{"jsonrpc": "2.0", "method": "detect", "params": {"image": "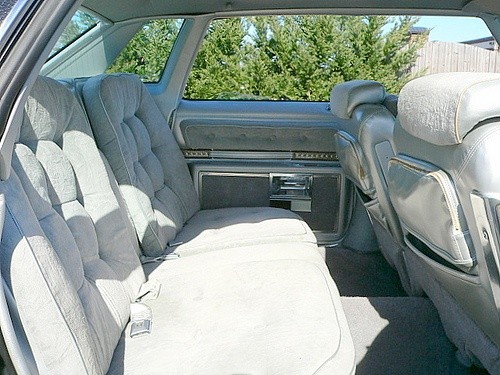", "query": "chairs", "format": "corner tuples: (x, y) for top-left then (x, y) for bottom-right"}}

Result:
(0, 74), (500, 375)
